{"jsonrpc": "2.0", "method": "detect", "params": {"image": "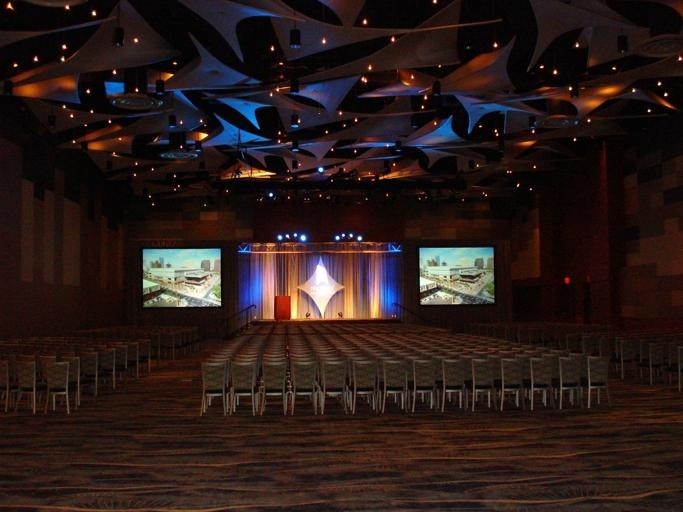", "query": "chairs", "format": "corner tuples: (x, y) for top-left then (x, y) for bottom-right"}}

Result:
(198, 319), (615, 417)
(2, 319), (201, 418)
(463, 319), (682, 393)
